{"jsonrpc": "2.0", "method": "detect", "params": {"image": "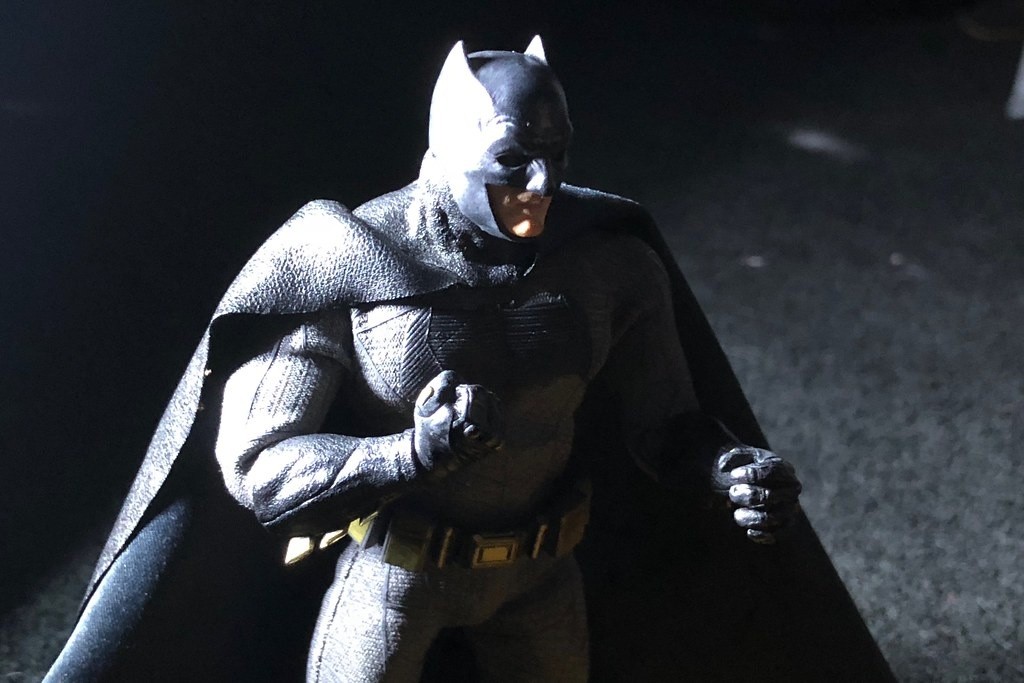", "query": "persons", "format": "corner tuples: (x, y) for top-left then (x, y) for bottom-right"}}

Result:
(41, 33), (899, 683)
